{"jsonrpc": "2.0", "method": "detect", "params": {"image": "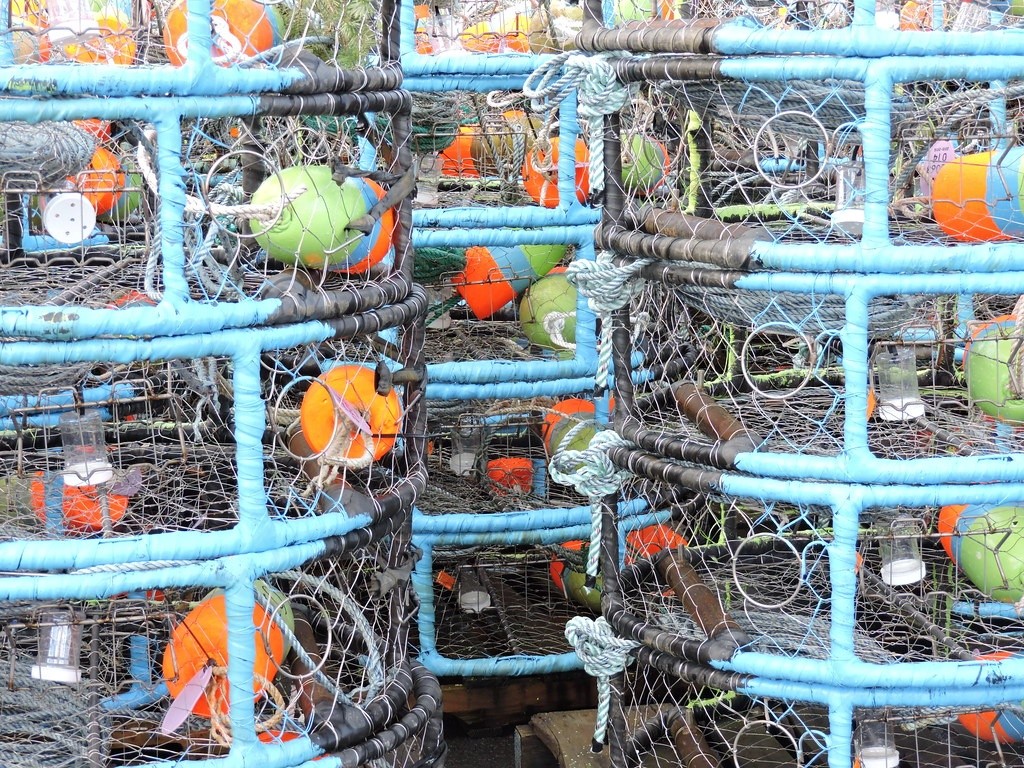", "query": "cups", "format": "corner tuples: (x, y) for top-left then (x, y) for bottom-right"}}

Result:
(425, 282), (452, 329)
(32, 609), (87, 683)
(61, 411), (112, 486)
(40, 179), (97, 243)
(875, 347), (924, 420)
(458, 565), (491, 616)
(427, 15), (464, 55)
(831, 161), (865, 233)
(45, 0), (100, 45)
(416, 153), (444, 204)
(447, 419), (483, 476)
(855, 707), (902, 768)
(874, 514), (926, 586)
